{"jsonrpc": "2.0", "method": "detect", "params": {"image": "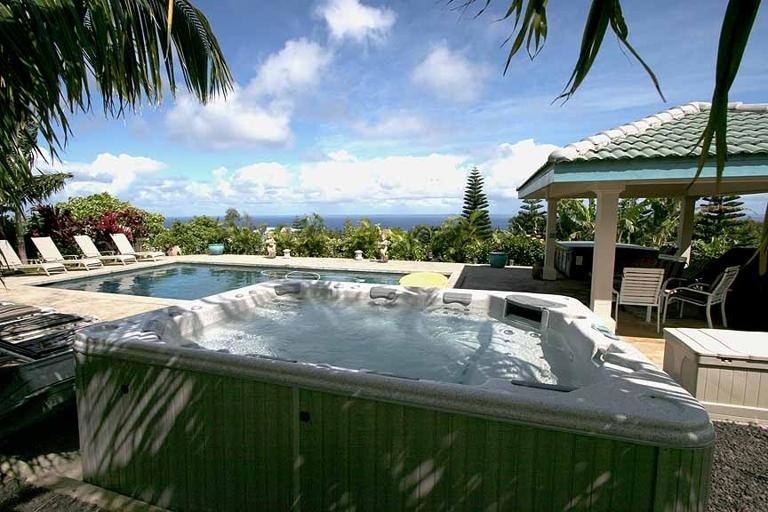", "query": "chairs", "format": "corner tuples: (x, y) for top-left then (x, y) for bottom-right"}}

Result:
(661, 266), (739, 329)
(31, 236), (105, 270)
(0, 240), (69, 276)
(73, 235), (138, 265)
(0, 300), (102, 370)
(662, 248), (761, 312)
(589, 267), (665, 333)
(110, 233), (166, 262)
